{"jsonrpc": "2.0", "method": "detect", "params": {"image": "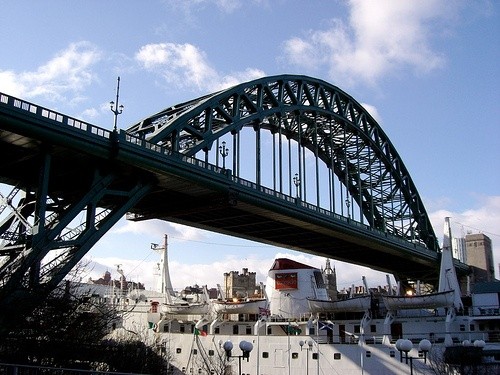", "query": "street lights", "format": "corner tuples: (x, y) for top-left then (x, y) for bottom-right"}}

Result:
(461, 338), (486, 375)
(110, 76), (124, 136)
(224, 339), (253, 375)
(219, 141), (229, 170)
(396, 338), (431, 375)
(80, 215), (500, 375)
(292, 173), (301, 199)
(300, 340), (313, 375)
